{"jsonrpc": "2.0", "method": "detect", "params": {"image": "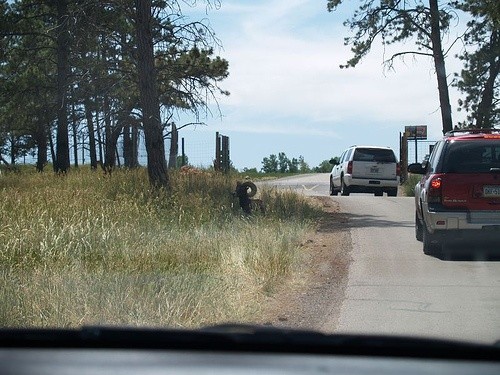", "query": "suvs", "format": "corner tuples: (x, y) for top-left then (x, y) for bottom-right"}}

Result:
(329, 145), (400, 197)
(405, 128), (500, 257)
